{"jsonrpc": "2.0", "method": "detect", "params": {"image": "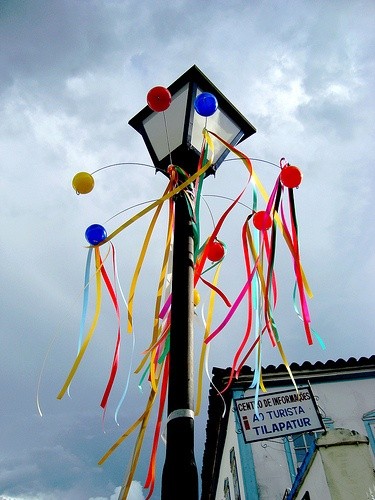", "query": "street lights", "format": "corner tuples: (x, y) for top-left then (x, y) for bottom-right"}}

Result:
(126, 65), (255, 500)
